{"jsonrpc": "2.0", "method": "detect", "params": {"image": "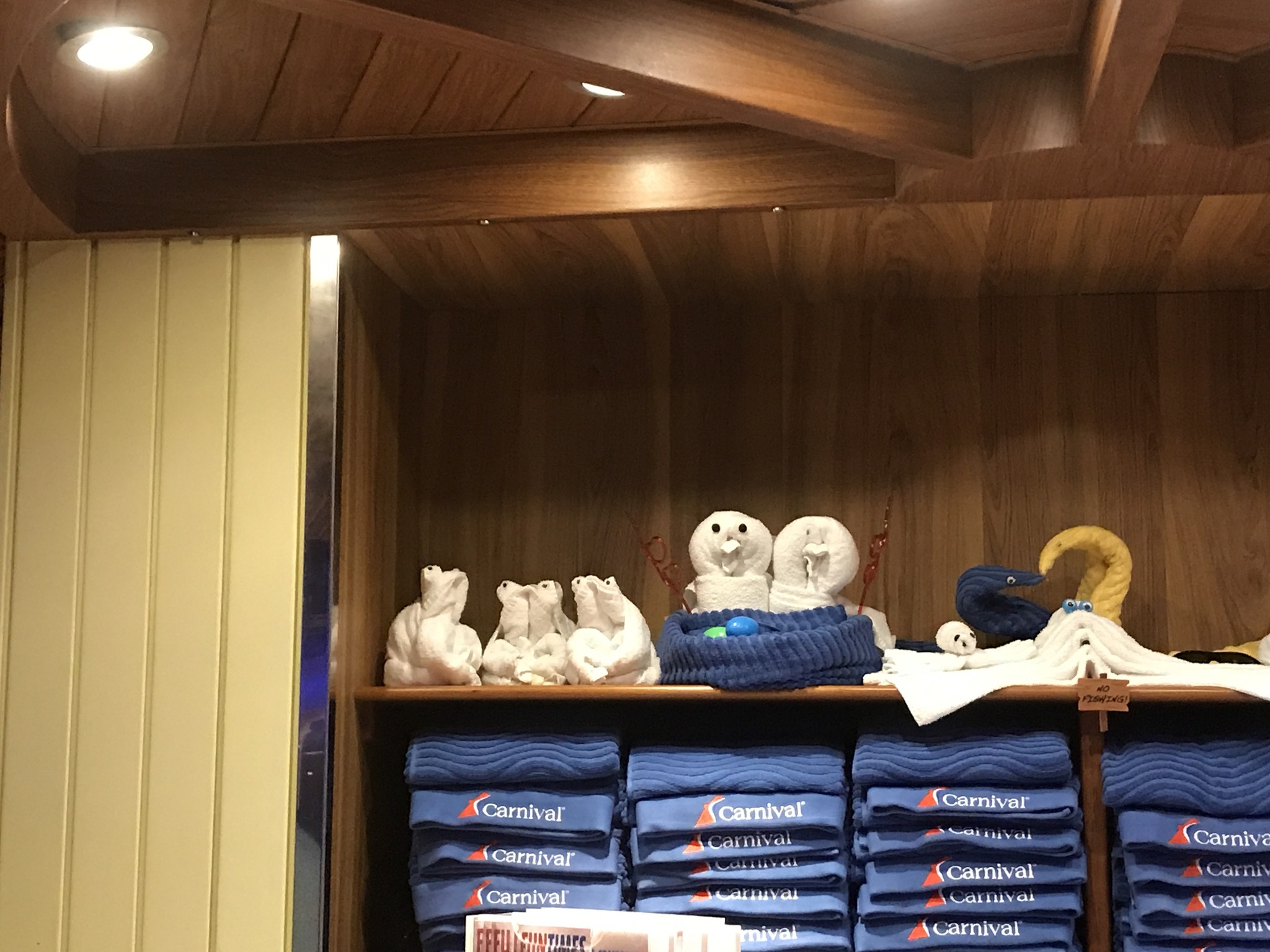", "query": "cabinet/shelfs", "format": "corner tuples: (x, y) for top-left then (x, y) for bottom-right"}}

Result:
(332, 197), (1270, 952)
